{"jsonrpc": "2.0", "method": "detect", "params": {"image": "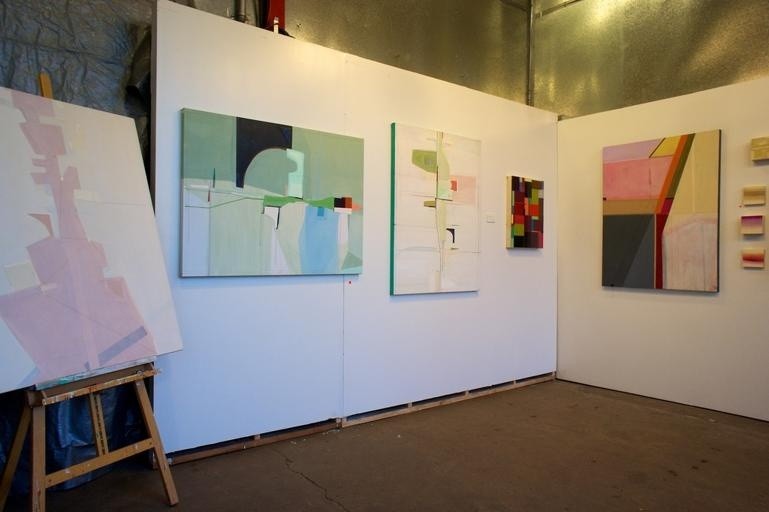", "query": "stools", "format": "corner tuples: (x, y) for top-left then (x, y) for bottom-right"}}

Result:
(1, 363), (180, 512)
